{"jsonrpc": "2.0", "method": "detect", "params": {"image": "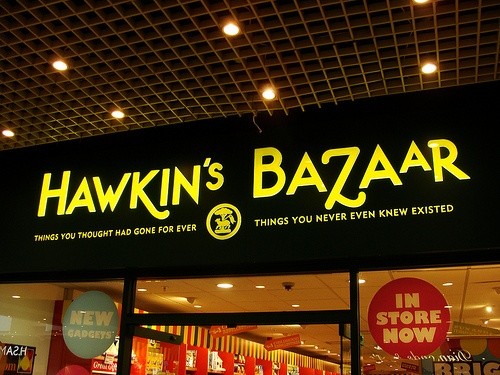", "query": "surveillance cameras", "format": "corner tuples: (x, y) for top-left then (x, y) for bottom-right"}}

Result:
(282, 282), (294, 292)
